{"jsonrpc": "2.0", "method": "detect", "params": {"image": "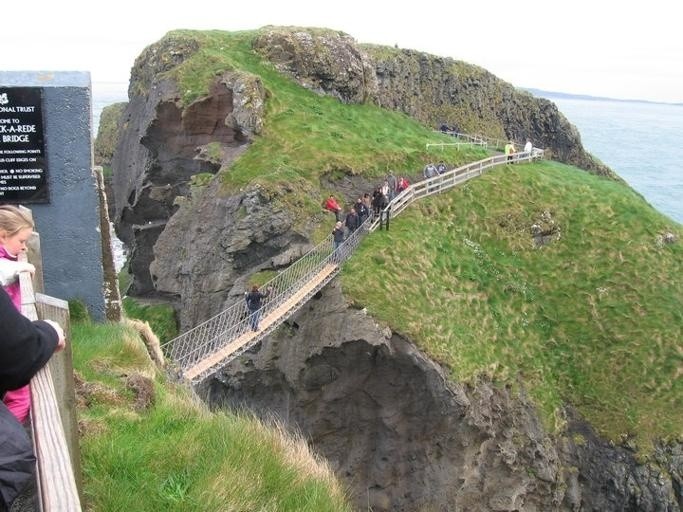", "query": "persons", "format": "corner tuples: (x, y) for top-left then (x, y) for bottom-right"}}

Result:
(524, 137), (533, 160)
(504, 140), (518, 164)
(244, 283), (273, 332)
(0, 282), (67, 512)
(423, 160), (440, 191)
(0, 203), (36, 422)
(436, 160), (448, 186)
(326, 170), (409, 248)
(440, 124), (462, 137)
